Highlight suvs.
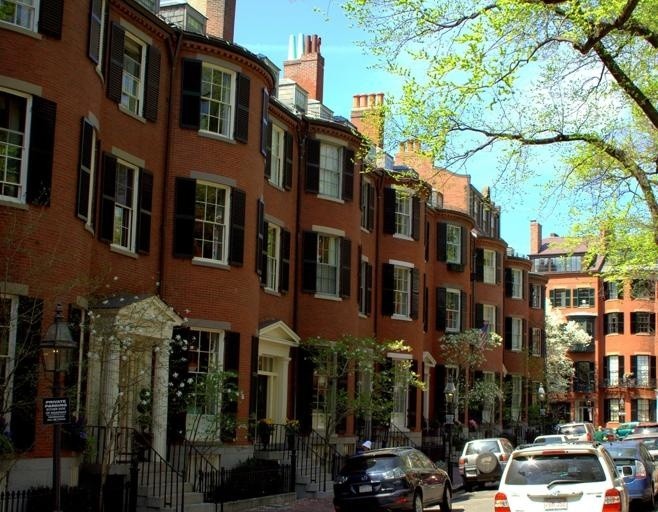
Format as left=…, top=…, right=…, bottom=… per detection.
left=492, top=440, right=633, bottom=512
left=456, top=436, right=518, bottom=494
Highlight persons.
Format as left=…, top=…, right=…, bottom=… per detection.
left=593, top=424, right=607, bottom=442
left=354, top=438, right=373, bottom=457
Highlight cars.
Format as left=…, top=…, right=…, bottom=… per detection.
left=528, top=417, right=658, bottom=508
left=330, top=443, right=456, bottom=512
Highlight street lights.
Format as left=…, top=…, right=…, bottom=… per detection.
left=584, top=383, right=595, bottom=422
left=443, top=367, right=457, bottom=485
left=537, top=381, right=547, bottom=434
left=33, top=301, right=82, bottom=512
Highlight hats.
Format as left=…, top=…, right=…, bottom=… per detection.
left=362, top=441, right=372, bottom=450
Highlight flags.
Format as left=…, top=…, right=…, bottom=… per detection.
left=476, top=322, right=489, bottom=352
